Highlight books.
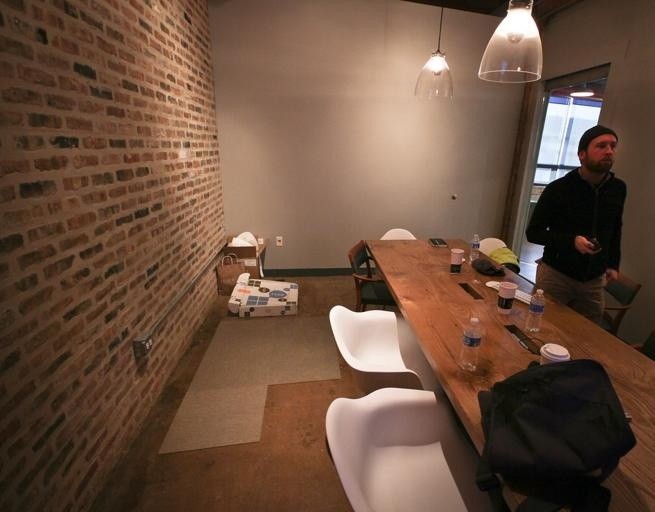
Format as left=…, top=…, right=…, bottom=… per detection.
left=429, top=238, right=448, bottom=247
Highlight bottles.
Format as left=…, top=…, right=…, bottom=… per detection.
left=525, top=289, right=545, bottom=332
left=470, top=234, right=480, bottom=261
left=459, top=317, right=481, bottom=371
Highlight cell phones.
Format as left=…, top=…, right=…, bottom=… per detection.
left=430, top=237, right=448, bottom=247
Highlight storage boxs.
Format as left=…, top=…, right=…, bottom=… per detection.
left=223, top=235, right=270, bottom=280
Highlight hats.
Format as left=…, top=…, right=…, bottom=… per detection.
left=578, top=125, right=618, bottom=156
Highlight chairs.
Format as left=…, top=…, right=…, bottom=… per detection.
left=379, top=227, right=417, bottom=240
left=479, top=237, right=507, bottom=256
left=630, top=329, right=654, bottom=360
left=329, top=304, right=442, bottom=393
left=603, top=271, right=642, bottom=335
left=348, top=239, right=397, bottom=312
left=325, top=388, right=508, bottom=511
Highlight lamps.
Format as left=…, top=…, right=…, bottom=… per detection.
left=478, top=0, right=543, bottom=84
left=414, top=0, right=454, bottom=100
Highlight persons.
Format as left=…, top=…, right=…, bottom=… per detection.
left=525, top=125, right=627, bottom=327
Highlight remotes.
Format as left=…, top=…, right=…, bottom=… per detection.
left=583, top=230, right=602, bottom=250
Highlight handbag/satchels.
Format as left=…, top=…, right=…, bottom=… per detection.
left=216, top=253, right=245, bottom=296
left=475, top=359, right=637, bottom=511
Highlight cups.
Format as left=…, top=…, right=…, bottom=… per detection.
left=497, top=282, right=518, bottom=315
left=449, top=248, right=464, bottom=273
left=539, top=343, right=570, bottom=366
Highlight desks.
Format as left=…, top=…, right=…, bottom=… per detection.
left=364, top=237, right=655, bottom=511
